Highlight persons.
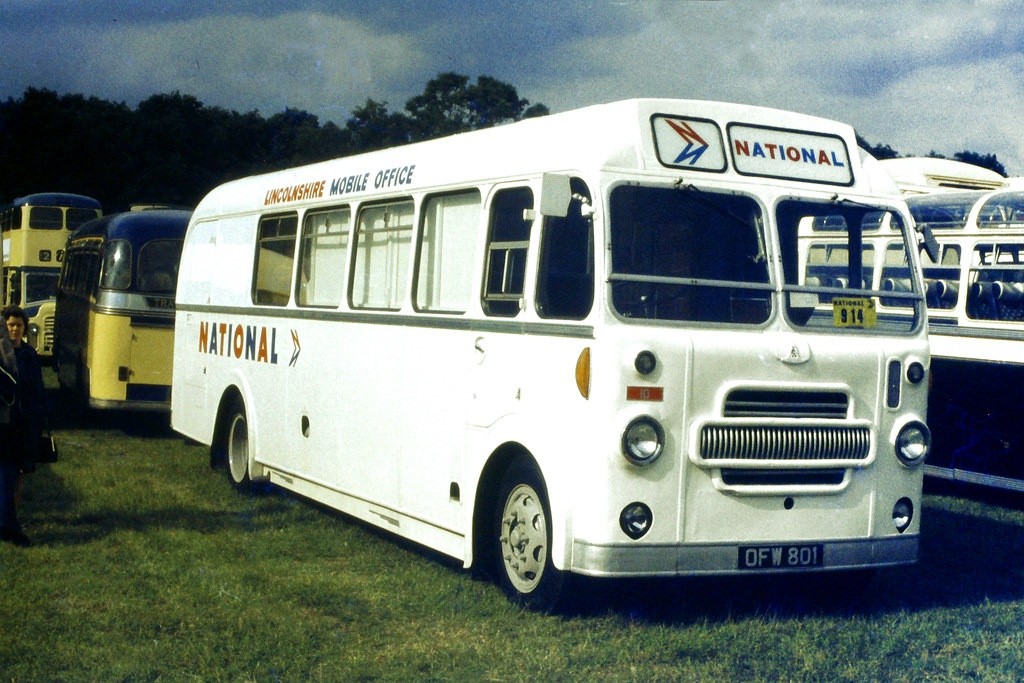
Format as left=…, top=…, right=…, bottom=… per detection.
left=0, top=306, right=58, bottom=548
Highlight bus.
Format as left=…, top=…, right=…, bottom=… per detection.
left=170, top=97, right=934, bottom=617
left=52, top=209, right=197, bottom=413
left=2, top=194, right=107, bottom=362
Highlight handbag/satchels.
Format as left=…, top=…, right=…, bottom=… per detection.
left=35, top=425, right=57, bottom=464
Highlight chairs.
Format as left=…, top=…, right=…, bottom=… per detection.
left=808, top=274, right=1024, bottom=324
left=142, top=270, right=174, bottom=291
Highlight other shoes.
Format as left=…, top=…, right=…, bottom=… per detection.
left=0, top=526, right=30, bottom=546
